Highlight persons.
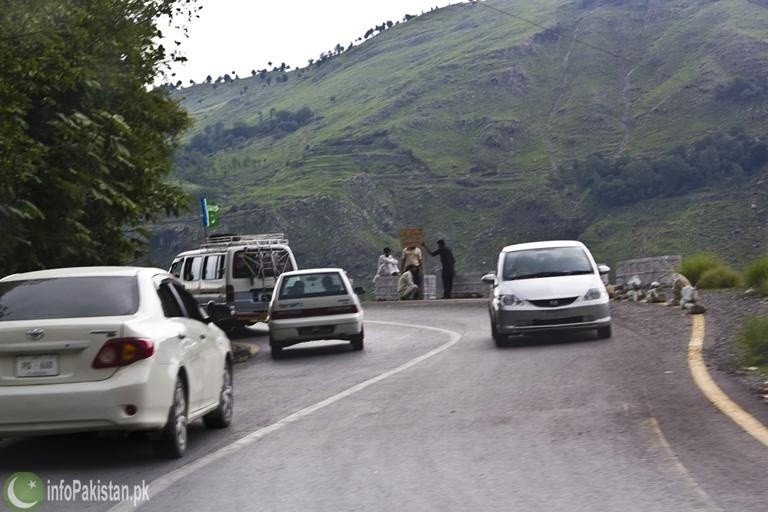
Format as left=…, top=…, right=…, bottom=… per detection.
left=421, top=240, right=456, bottom=299
left=378, top=247, right=401, bottom=275
left=397, top=247, right=424, bottom=300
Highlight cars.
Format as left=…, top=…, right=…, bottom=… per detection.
left=264, top=267, right=366, bottom=354
left=1, top=266, right=238, bottom=459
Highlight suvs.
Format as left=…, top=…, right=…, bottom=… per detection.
left=480, top=238, right=615, bottom=344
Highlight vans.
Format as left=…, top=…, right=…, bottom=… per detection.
left=168, top=233, right=299, bottom=335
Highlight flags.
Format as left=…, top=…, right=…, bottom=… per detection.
left=201, top=196, right=219, bottom=229
